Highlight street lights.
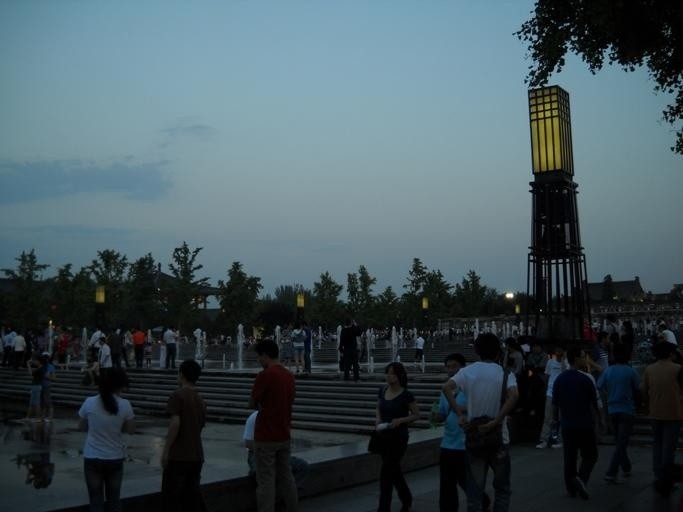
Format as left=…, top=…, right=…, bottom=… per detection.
left=297, top=290, right=305, bottom=326
left=420, top=297, right=431, bottom=334
left=93, top=283, right=106, bottom=330
left=515, top=302, right=521, bottom=328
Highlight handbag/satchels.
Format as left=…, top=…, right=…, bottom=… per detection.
left=464, top=416, right=502, bottom=447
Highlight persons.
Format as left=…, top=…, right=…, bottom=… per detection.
left=499, top=300, right=682, bottom=449
left=643, top=340, right=682, bottom=491
left=17, top=419, right=55, bottom=489
left=163, top=323, right=179, bottom=369
left=337, top=320, right=362, bottom=381
left=249, top=339, right=298, bottom=510
left=2, top=327, right=39, bottom=370
left=82, top=326, right=153, bottom=384
left=25, top=354, right=56, bottom=419
left=77, top=368, right=136, bottom=509
left=597, top=343, right=640, bottom=481
left=415, top=333, right=425, bottom=360
left=403, top=328, right=464, bottom=347
left=162, top=359, right=206, bottom=510
left=280, top=320, right=313, bottom=376
left=51, top=325, right=81, bottom=371
left=384, top=326, right=391, bottom=348
left=551, top=346, right=600, bottom=498
left=243, top=410, right=308, bottom=487
left=443, top=332, right=518, bottom=511
left=431, top=353, right=492, bottom=511
left=376, top=362, right=419, bottom=510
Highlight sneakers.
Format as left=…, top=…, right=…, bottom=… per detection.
left=605, top=469, right=631, bottom=480
left=567, top=475, right=589, bottom=498
left=536, top=435, right=561, bottom=448
